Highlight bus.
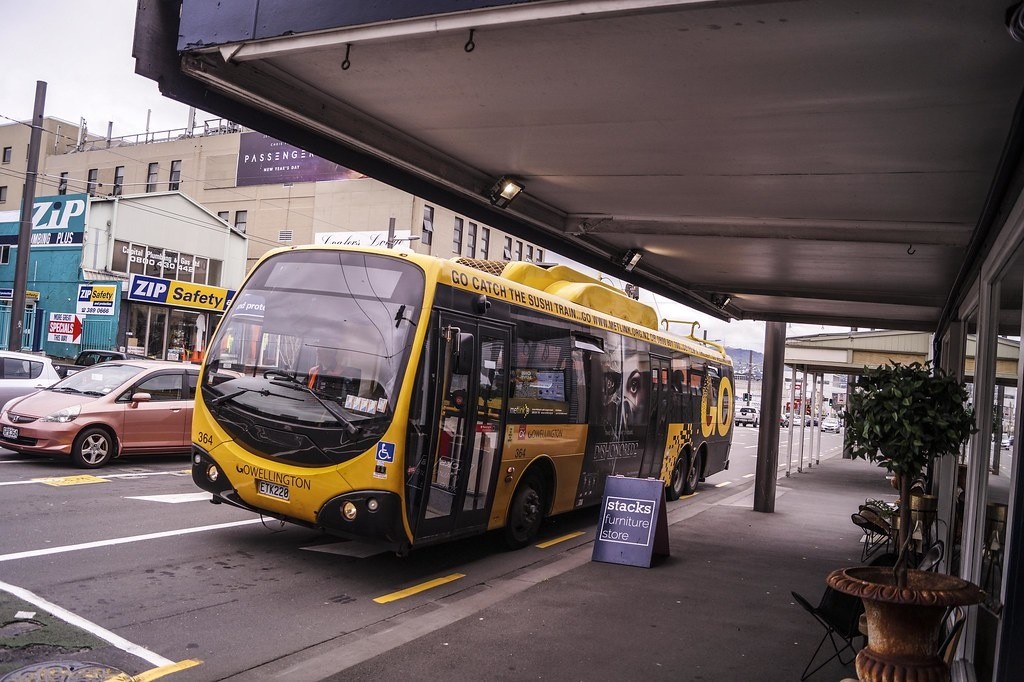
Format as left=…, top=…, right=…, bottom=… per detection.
left=190, top=243, right=738, bottom=560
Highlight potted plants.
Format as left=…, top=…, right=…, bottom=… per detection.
left=826, top=357, right=981, bottom=682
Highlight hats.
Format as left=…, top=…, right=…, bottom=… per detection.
left=314, top=337, right=339, bottom=347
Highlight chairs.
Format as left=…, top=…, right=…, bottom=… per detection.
left=340, top=364, right=360, bottom=399
left=156, top=377, right=177, bottom=400
left=792, top=540, right=966, bottom=682
left=851, top=498, right=892, bottom=563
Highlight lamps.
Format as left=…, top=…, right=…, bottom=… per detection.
left=712, top=292, right=731, bottom=309
left=476, top=176, right=526, bottom=210
left=611, top=249, right=643, bottom=272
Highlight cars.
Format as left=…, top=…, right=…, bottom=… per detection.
left=780, top=414, right=790, bottom=428
left=806, top=417, right=823, bottom=427
left=0, top=350, right=61, bottom=414
left=0, top=359, right=247, bottom=468
left=821, top=417, right=841, bottom=433
left=785, top=413, right=797, bottom=419
left=793, top=415, right=811, bottom=426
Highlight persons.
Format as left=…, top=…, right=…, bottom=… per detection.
left=602, top=332, right=650, bottom=426
left=301, top=334, right=356, bottom=399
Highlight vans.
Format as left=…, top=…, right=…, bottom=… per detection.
left=1001, top=433, right=1010, bottom=448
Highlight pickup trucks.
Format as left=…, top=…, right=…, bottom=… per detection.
left=52, top=349, right=157, bottom=380
left=734, top=407, right=760, bottom=427
